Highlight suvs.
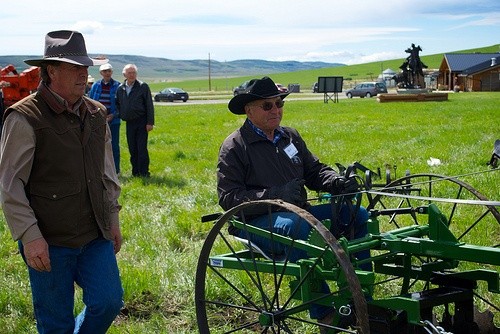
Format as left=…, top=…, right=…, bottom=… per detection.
left=346, top=82, right=388, bottom=99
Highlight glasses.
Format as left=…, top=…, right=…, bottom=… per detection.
left=250, top=99, right=286, bottom=111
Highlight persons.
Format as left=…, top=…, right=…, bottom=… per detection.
left=405, top=44, right=422, bottom=74
left=88, top=63, right=121, bottom=177
left=0, top=29, right=123, bottom=334
left=114, top=63, right=155, bottom=178
left=217, top=76, right=373, bottom=334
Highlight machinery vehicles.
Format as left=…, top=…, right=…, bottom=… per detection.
left=0, top=65, right=43, bottom=118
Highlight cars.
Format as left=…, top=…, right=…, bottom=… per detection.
left=311, top=82, right=319, bottom=93
left=154, top=88, right=189, bottom=102
left=275, top=83, right=289, bottom=92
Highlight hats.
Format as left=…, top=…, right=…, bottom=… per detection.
left=99, top=63, right=113, bottom=72
left=228, top=76, right=290, bottom=116
left=24, top=29, right=95, bottom=66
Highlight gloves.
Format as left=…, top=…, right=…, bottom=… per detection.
left=270, top=178, right=305, bottom=203
left=335, top=174, right=358, bottom=199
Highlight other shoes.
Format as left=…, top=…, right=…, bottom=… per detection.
left=319, top=309, right=352, bottom=334
